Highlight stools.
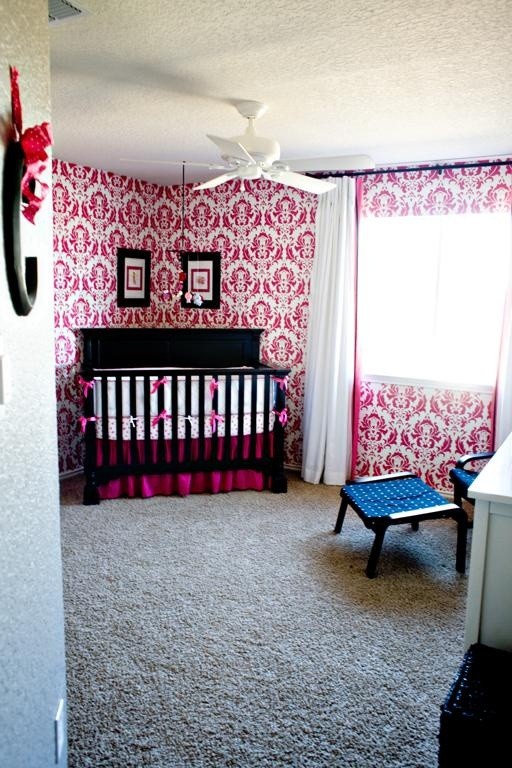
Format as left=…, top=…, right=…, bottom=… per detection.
left=334, top=471, right=468, bottom=578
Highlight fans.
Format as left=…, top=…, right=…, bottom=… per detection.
left=190, top=97, right=337, bottom=196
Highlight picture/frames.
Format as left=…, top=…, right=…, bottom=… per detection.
left=116, top=246, right=152, bottom=308
left=181, top=252, right=221, bottom=309
